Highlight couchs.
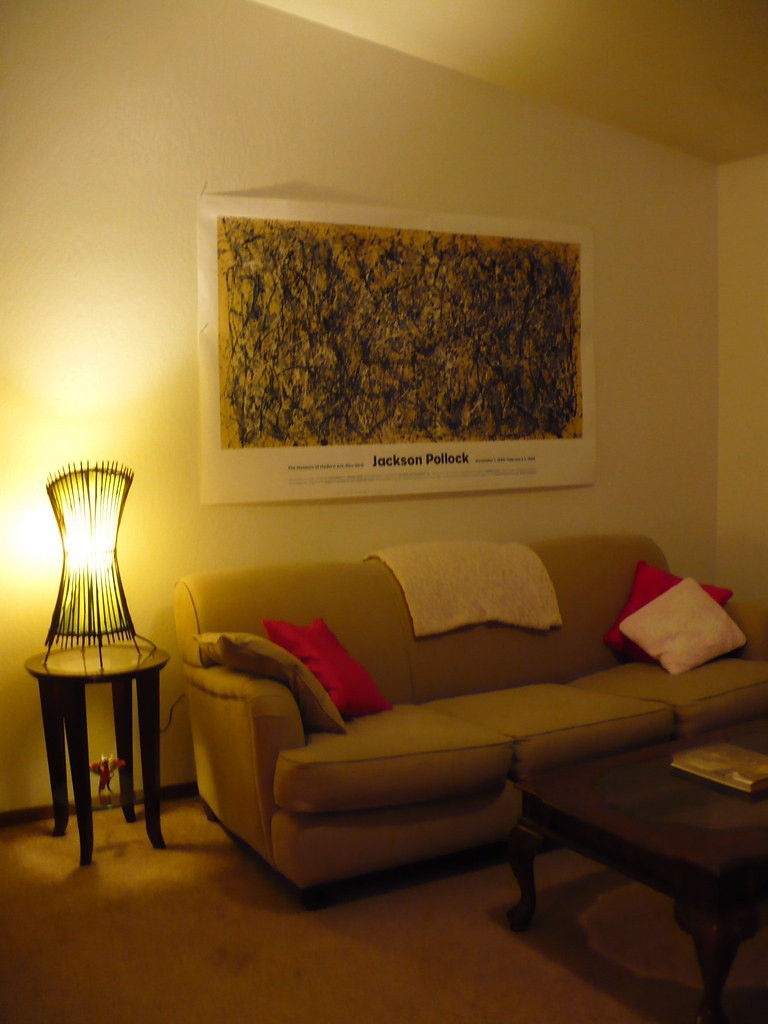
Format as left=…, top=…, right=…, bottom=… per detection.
left=167, top=532, right=768, bottom=909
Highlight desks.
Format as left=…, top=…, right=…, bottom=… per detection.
left=25, top=643, right=171, bottom=867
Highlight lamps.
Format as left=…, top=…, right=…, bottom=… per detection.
left=43, top=463, right=140, bottom=669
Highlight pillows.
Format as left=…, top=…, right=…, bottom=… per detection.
left=193, top=631, right=347, bottom=732
left=260, top=617, right=393, bottom=715
left=606, top=560, right=734, bottom=663
left=619, top=577, right=746, bottom=676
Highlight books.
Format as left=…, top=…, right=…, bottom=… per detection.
left=671, top=744, right=768, bottom=793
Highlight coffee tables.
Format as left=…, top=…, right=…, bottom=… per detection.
left=509, top=718, right=768, bottom=1023
